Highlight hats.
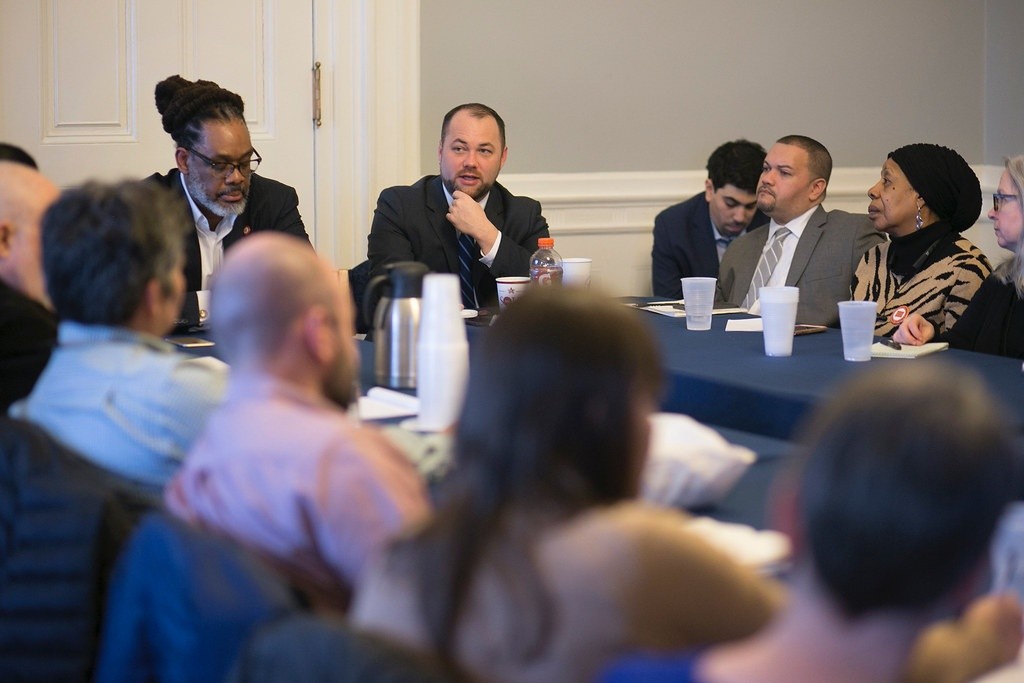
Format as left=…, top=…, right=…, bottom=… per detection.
left=887, top=143, right=982, bottom=233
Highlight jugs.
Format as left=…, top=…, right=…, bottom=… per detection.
left=361, top=259, right=430, bottom=389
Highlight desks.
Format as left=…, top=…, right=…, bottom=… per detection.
left=161, top=295, right=1024, bottom=536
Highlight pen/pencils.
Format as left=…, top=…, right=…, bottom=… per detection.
left=635, top=303, right=679, bottom=306
left=878, top=338, right=903, bottom=351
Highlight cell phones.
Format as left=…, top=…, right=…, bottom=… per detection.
left=464, top=317, right=490, bottom=326
left=165, top=337, right=215, bottom=347
left=793, top=324, right=827, bottom=335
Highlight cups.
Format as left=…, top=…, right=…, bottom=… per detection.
left=416, top=273, right=471, bottom=427
left=681, top=277, right=717, bottom=331
left=758, top=284, right=800, bottom=358
left=559, top=258, right=592, bottom=290
left=838, top=301, right=878, bottom=362
left=196, top=290, right=211, bottom=323
left=496, top=276, right=531, bottom=310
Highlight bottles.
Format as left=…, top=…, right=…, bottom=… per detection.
left=530, top=238, right=564, bottom=289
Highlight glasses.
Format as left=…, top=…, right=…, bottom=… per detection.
left=993, top=193, right=1024, bottom=211
left=182, top=143, right=263, bottom=176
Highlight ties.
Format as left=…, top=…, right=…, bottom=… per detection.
left=741, top=226, right=792, bottom=311
left=458, top=228, right=478, bottom=309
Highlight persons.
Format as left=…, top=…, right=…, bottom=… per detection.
left=850, top=143, right=993, bottom=338
left=7, top=179, right=231, bottom=487
left=164, top=231, right=436, bottom=632
left=651, top=139, right=771, bottom=301
left=342, top=279, right=1024, bottom=683
left=578, top=359, right=1024, bottom=683
left=713, top=135, right=888, bottom=328
left=142, top=75, right=317, bottom=334
left=367, top=103, right=550, bottom=310
left=0, top=143, right=64, bottom=413
left=892, top=155, right=1024, bottom=361
